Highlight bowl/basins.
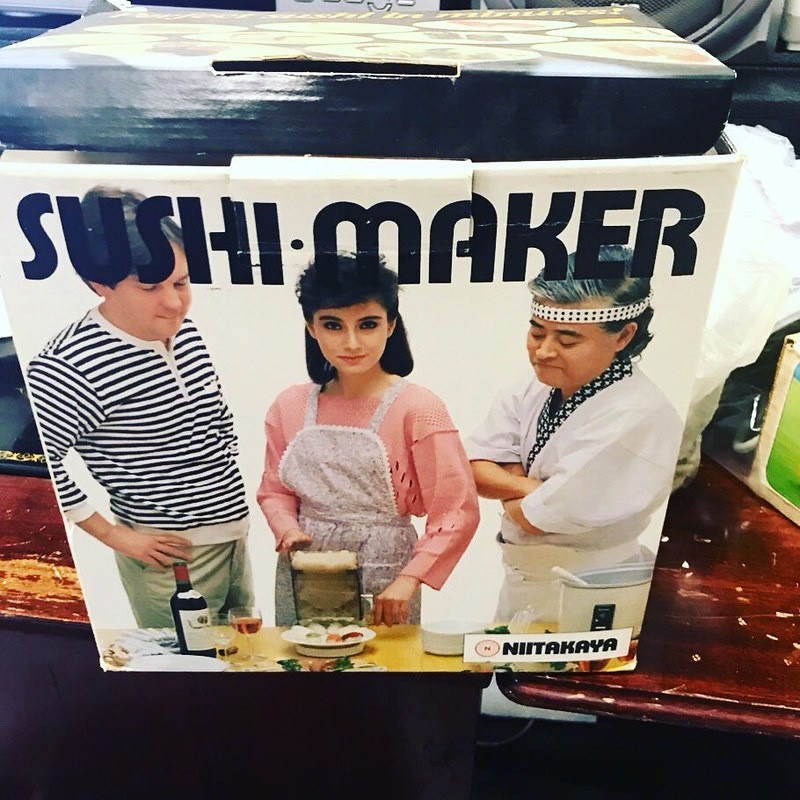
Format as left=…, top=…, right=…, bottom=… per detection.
left=420, top=620, right=488, bottom=656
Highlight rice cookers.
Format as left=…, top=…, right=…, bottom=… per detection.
left=558, top=561, right=656, bottom=631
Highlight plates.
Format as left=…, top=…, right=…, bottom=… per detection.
left=281, top=629, right=377, bottom=658
left=128, top=655, right=227, bottom=672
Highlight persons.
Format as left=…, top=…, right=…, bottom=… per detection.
left=463, top=247, right=679, bottom=625
left=25, top=184, right=257, bottom=629
left=257, top=254, right=481, bottom=627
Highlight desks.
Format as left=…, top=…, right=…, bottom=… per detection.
left=0, top=473, right=492, bottom=691
left=495, top=453, right=800, bottom=742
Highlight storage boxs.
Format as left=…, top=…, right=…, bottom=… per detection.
left=0, top=4, right=800, bottom=672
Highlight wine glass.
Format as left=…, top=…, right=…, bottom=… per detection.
left=203, top=613, right=244, bottom=667
left=228, top=606, right=268, bottom=662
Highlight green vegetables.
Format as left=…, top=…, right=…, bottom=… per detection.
left=484, top=626, right=569, bottom=671
left=277, top=656, right=354, bottom=672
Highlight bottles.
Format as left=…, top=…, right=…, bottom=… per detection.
left=169, top=561, right=217, bottom=659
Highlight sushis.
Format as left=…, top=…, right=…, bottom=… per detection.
left=306, top=631, right=363, bottom=644
